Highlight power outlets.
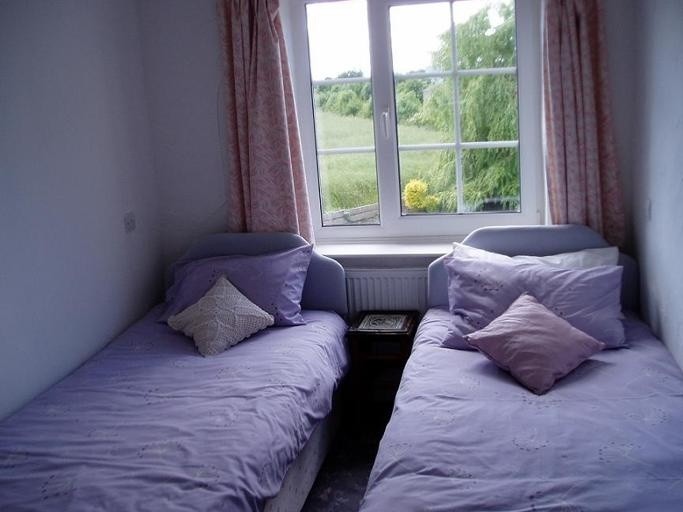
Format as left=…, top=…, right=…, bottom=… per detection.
left=124, top=212, right=136, bottom=234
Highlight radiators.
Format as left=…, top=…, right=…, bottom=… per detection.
left=346, top=266, right=428, bottom=313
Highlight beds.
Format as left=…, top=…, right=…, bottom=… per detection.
left=359, top=223, right=683, bottom=512
left=0, top=232, right=348, bottom=512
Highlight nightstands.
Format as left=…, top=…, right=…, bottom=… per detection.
left=347, top=309, right=420, bottom=408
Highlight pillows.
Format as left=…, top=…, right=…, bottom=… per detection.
left=157, top=243, right=313, bottom=327
left=441, top=256, right=628, bottom=348
left=462, top=290, right=605, bottom=395
left=167, top=276, right=275, bottom=359
left=452, top=241, right=619, bottom=267
